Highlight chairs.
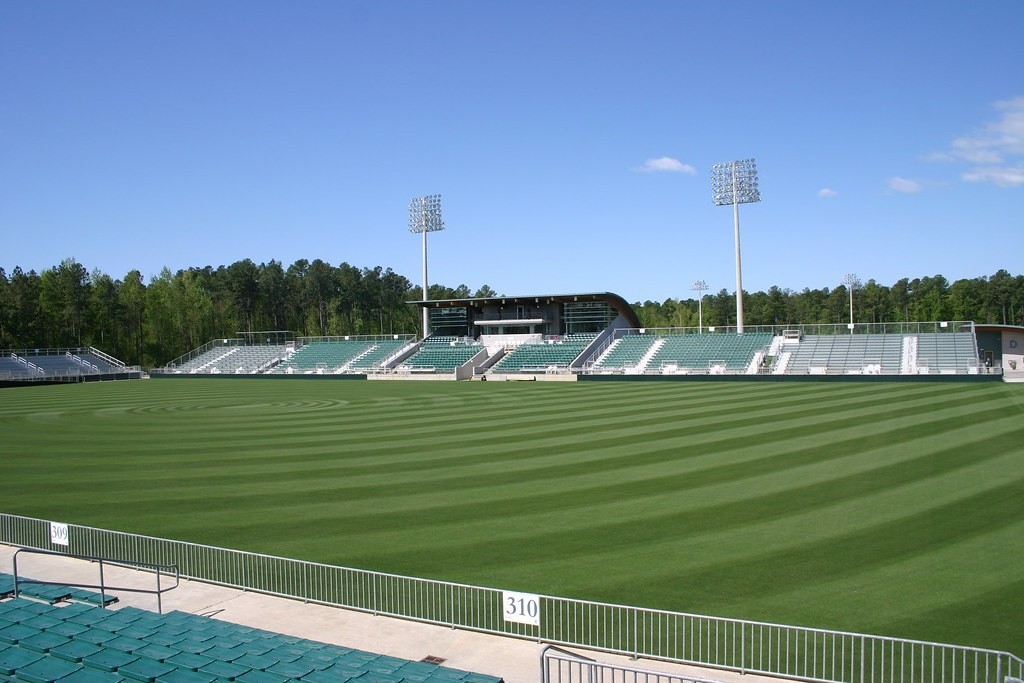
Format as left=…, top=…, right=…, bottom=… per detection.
left=165, top=330, right=775, bottom=379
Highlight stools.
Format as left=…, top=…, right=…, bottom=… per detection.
left=0, top=573, right=504, bottom=683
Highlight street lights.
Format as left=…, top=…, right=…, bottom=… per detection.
left=841, top=270, right=863, bottom=333
left=689, top=280, right=711, bottom=335
left=407, top=194, right=446, bottom=337
left=711, top=157, right=763, bottom=334
